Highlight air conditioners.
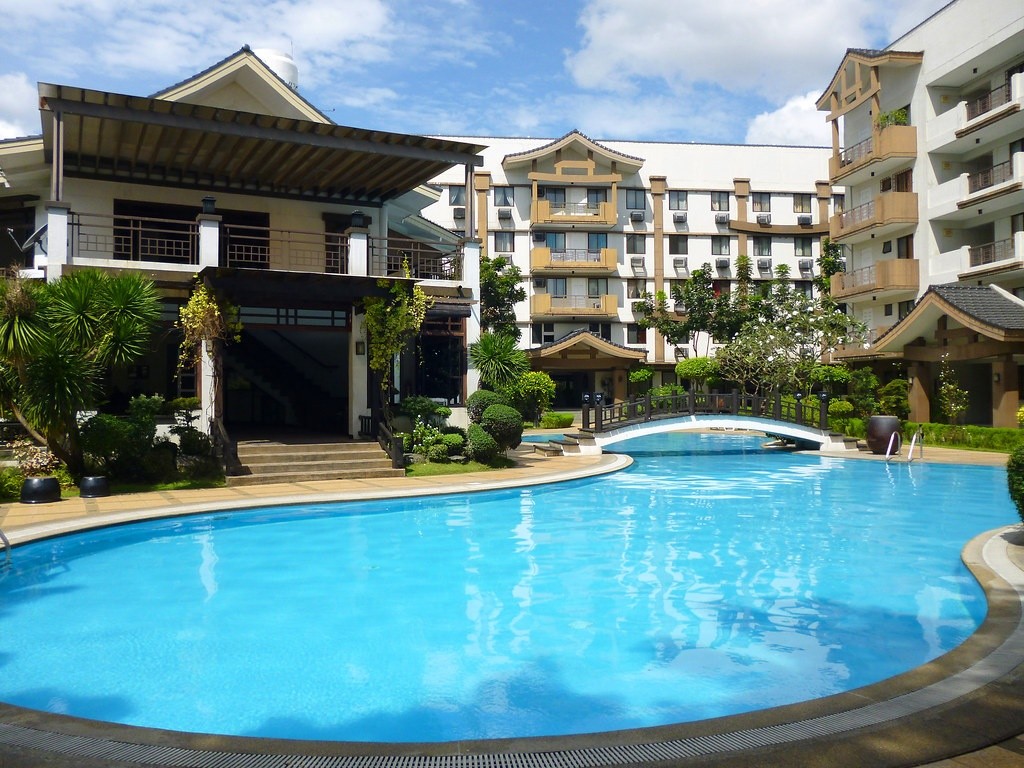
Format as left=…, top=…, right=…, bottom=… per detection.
left=673, top=257, right=687, bottom=268
left=799, top=258, right=813, bottom=269
left=630, top=210, right=644, bottom=222
left=533, top=278, right=545, bottom=287
left=715, top=212, right=730, bottom=224
left=757, top=214, right=771, bottom=223
left=454, top=207, right=465, bottom=219
left=499, top=254, right=512, bottom=265
left=673, top=212, right=687, bottom=223
left=498, top=208, right=511, bottom=220
left=631, top=256, right=645, bottom=267
left=532, top=232, right=545, bottom=242
left=757, top=258, right=772, bottom=269
left=715, top=257, right=730, bottom=268
left=797, top=215, right=812, bottom=225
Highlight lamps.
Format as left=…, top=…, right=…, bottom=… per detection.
left=355, top=341, right=366, bottom=356
